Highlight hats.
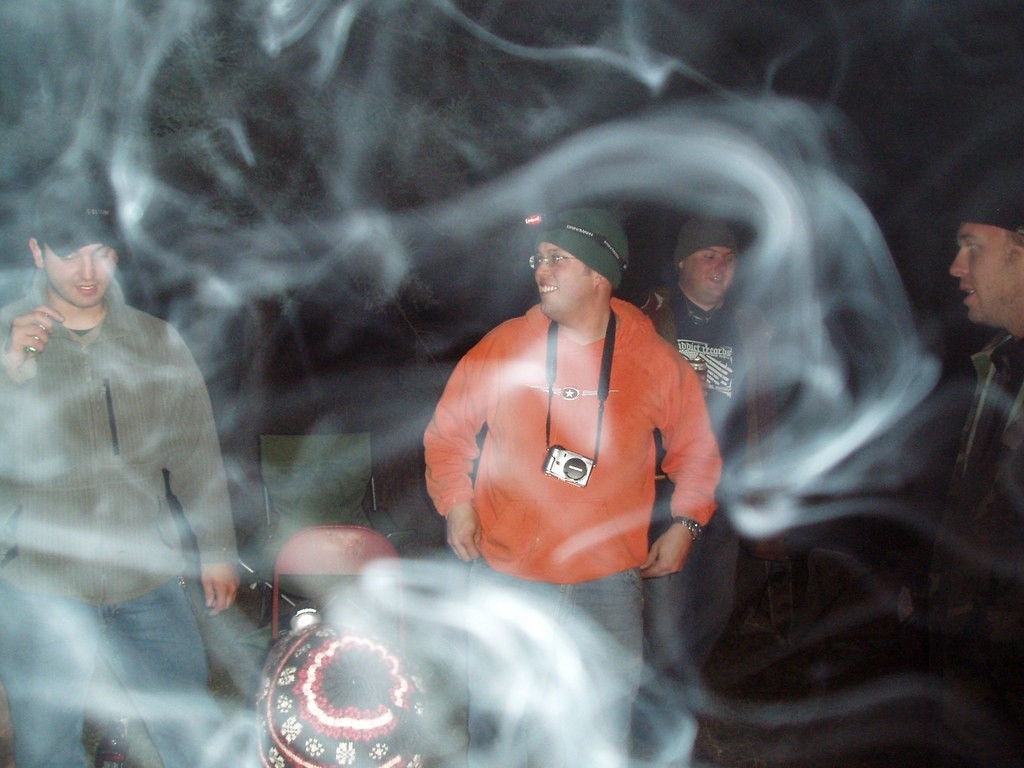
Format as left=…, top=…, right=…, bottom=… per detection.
left=671, top=215, right=738, bottom=266
left=31, top=178, right=126, bottom=257
left=951, top=174, right=1024, bottom=237
left=532, top=207, right=630, bottom=291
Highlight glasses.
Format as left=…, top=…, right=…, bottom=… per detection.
left=528, top=252, right=577, bottom=268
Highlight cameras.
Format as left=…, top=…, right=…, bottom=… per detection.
left=544, top=445, right=594, bottom=488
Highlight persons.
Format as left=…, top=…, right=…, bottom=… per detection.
left=251, top=158, right=1024, bottom=768
left=1, top=178, right=242, bottom=768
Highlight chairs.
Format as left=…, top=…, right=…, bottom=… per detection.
left=260, top=432, right=435, bottom=768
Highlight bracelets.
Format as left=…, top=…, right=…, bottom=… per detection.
left=671, top=516, right=704, bottom=542
